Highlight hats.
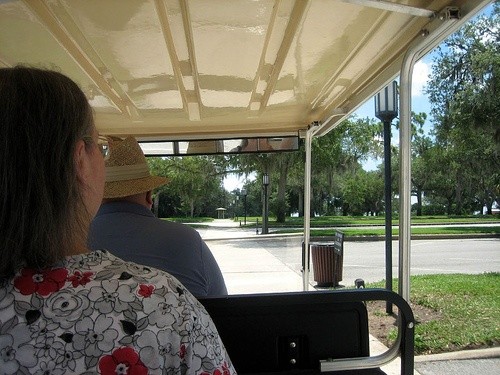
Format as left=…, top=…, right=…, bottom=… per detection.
left=101, top=136, right=171, bottom=200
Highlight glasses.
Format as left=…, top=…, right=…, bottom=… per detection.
left=80, top=136, right=114, bottom=160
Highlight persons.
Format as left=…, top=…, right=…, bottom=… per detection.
left=0, top=64, right=237, bottom=375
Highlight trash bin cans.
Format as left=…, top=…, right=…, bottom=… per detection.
left=311, top=242, right=343, bottom=288
